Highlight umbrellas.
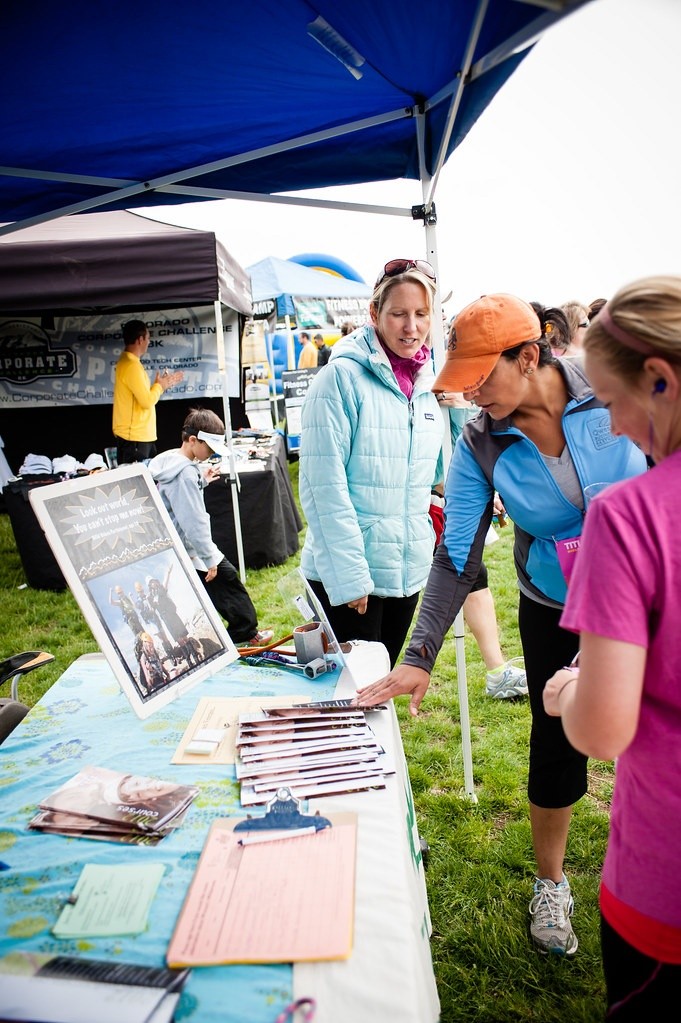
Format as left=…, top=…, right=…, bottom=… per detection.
left=244, top=252, right=369, bottom=370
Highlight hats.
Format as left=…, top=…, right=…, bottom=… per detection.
left=84, top=453, right=108, bottom=471
left=16, top=453, right=90, bottom=485
left=145, top=576, right=159, bottom=589
left=141, top=633, right=153, bottom=642
left=431, top=293, right=542, bottom=396
left=135, top=582, right=143, bottom=591
left=184, top=426, right=231, bottom=456
left=115, top=586, right=123, bottom=594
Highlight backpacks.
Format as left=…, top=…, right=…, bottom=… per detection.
left=140, top=649, right=164, bottom=687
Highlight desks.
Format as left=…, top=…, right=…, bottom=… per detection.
left=2, top=430, right=303, bottom=593
left=0, top=641, right=441, bottom=1023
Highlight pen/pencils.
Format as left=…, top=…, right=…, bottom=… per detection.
left=237, top=825, right=327, bottom=846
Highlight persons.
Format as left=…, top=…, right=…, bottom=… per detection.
left=545, top=276, right=681, bottom=1022
left=113, top=321, right=185, bottom=466
left=104, top=770, right=182, bottom=805
left=295, top=298, right=612, bottom=698
left=352, top=294, right=653, bottom=953
left=148, top=409, right=274, bottom=646
left=109, top=563, right=203, bottom=690
left=298, top=260, right=452, bottom=671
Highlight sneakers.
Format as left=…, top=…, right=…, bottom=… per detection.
left=234, top=628, right=274, bottom=646
left=532, top=871, right=579, bottom=954
left=483, top=664, right=530, bottom=699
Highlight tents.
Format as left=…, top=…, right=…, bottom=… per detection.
left=1, top=0, right=595, bottom=803
left=1, top=211, right=252, bottom=586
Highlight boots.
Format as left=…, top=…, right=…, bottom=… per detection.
left=180, top=641, right=198, bottom=667
left=161, top=641, right=176, bottom=667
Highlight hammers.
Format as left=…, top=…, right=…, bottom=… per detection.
left=261, top=656, right=326, bottom=679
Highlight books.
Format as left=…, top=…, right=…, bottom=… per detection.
left=25, top=766, right=202, bottom=846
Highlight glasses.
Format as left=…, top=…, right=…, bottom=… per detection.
left=577, top=319, right=590, bottom=327
left=373, top=259, right=437, bottom=289
